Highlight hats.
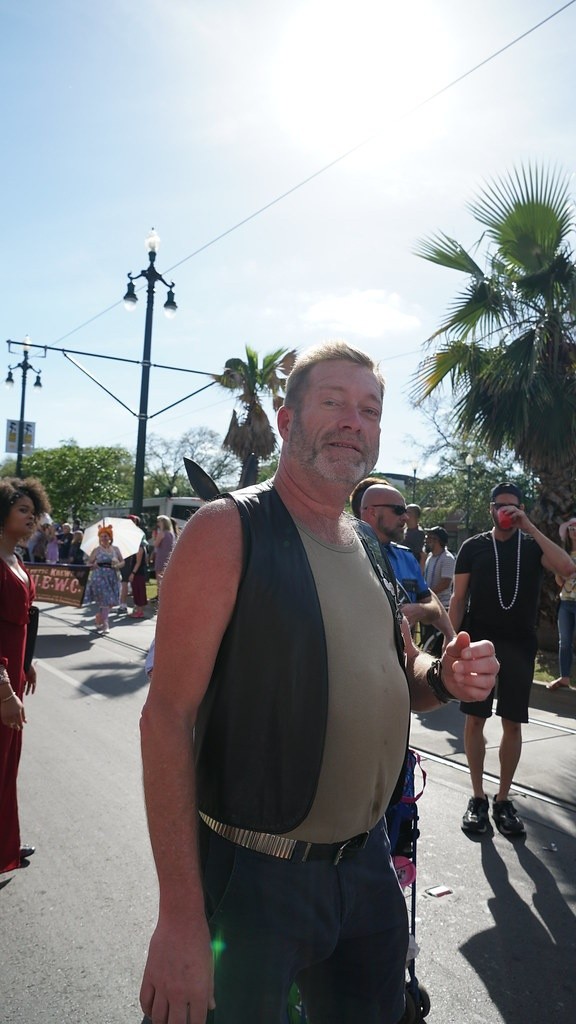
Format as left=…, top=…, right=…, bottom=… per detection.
left=97, top=524, right=114, bottom=546
left=559, top=517, right=576, bottom=541
left=424, top=525, right=447, bottom=543
left=493, top=482, right=521, bottom=502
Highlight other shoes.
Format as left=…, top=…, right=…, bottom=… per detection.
left=20, top=845, right=35, bottom=857
left=129, top=611, right=145, bottom=618
left=104, top=621, right=109, bottom=633
left=115, top=607, right=128, bottom=613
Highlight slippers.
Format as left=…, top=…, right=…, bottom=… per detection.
left=546, top=676, right=568, bottom=689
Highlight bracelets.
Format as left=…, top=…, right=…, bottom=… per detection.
left=2, top=692, right=16, bottom=702
left=426, top=659, right=457, bottom=705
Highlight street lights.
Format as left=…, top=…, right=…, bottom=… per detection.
left=121, top=224, right=179, bottom=517
left=411, top=456, right=420, bottom=505
left=3, top=335, right=43, bottom=480
left=464, top=451, right=475, bottom=544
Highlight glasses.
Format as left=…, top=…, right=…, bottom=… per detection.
left=364, top=505, right=407, bottom=515
left=567, top=525, right=576, bottom=531
left=494, top=503, right=520, bottom=510
left=424, top=534, right=440, bottom=541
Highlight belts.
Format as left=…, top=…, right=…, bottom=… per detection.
left=200, top=809, right=371, bottom=866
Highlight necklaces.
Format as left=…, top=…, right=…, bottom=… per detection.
left=492, top=527, right=521, bottom=609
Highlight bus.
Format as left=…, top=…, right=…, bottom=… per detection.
left=85, top=496, right=210, bottom=547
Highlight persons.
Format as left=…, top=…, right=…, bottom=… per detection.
left=135, top=345, right=503, bottom=1023
left=545, top=517, right=575, bottom=690
left=83, top=524, right=126, bottom=634
left=362, top=486, right=441, bottom=626
left=350, top=478, right=458, bottom=649
left=23, top=515, right=175, bottom=619
left=0, top=475, right=50, bottom=871
left=442, top=481, right=576, bottom=840
left=404, top=504, right=425, bottom=563
left=420, top=525, right=455, bottom=658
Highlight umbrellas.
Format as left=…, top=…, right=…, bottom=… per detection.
left=79, top=514, right=146, bottom=560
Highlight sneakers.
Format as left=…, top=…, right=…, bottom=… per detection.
left=461, top=795, right=488, bottom=833
left=492, top=794, right=524, bottom=835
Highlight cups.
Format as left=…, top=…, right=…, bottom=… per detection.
left=498, top=506, right=513, bottom=529
